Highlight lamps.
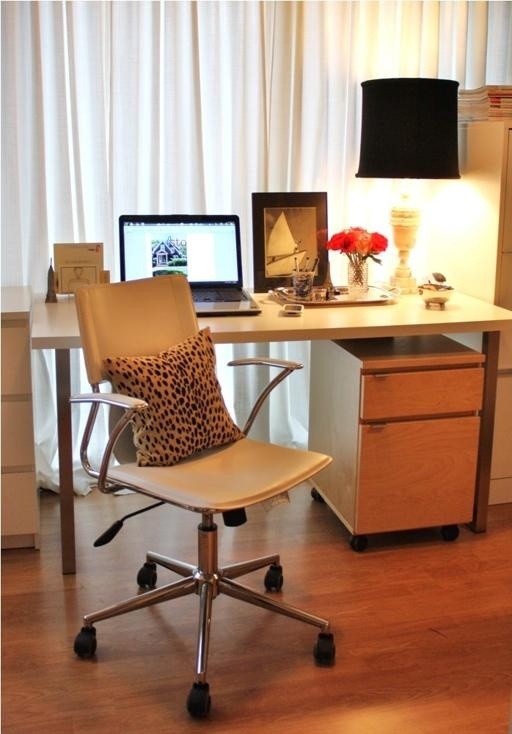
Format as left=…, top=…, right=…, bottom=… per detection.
left=350, top=73, right=463, bottom=300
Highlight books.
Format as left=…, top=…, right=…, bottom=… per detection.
left=458, top=85, right=512, bottom=127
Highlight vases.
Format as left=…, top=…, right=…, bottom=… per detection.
left=344, top=248, right=373, bottom=295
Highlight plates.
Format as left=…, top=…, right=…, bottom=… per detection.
left=274, top=287, right=397, bottom=304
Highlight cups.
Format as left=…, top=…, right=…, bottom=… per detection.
left=291, top=268, right=314, bottom=301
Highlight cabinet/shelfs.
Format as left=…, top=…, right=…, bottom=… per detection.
left=449, top=114, right=512, bottom=512
left=2, top=280, right=45, bottom=556
left=300, top=339, right=484, bottom=555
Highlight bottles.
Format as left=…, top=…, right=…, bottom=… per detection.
left=45, top=265, right=59, bottom=304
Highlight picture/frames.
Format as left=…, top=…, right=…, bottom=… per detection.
left=249, top=191, right=329, bottom=294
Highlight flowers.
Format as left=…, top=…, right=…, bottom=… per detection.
left=325, top=224, right=389, bottom=286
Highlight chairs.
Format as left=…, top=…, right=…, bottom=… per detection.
left=61, top=269, right=340, bottom=719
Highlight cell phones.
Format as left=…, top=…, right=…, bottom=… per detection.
left=282, top=304, right=304, bottom=317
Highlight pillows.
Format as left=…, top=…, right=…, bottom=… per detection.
left=102, top=321, right=246, bottom=469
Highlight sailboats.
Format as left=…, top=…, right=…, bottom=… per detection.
left=264, top=208, right=309, bottom=277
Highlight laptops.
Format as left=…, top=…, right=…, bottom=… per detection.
left=119, top=215, right=261, bottom=316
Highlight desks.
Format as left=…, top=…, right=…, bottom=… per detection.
left=27, top=283, right=512, bottom=581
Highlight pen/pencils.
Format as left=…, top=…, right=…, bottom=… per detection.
left=294, top=253, right=319, bottom=272
